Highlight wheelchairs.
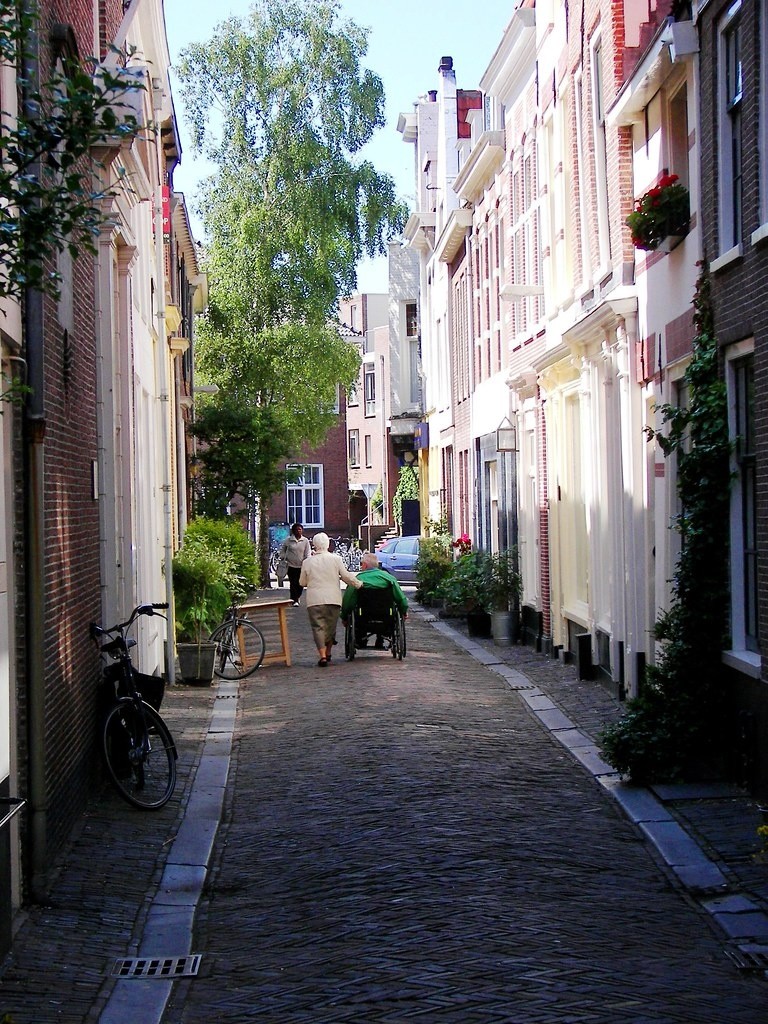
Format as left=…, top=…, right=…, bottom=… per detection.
left=345, top=586, right=406, bottom=661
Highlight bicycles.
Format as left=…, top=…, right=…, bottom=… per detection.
left=90, top=602, right=179, bottom=811
left=208, top=604, right=266, bottom=680
left=268, top=535, right=363, bottom=577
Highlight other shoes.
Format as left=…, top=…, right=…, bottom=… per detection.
left=293, top=602, right=299, bottom=607
left=375, top=642, right=383, bottom=647
left=354, top=638, right=367, bottom=648
left=298, top=597, right=301, bottom=604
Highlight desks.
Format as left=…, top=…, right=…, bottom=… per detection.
left=230, top=597, right=295, bottom=673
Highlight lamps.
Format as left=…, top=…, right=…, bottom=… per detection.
left=496, top=416, right=519, bottom=452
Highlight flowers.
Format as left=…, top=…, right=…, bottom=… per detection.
left=625, top=174, right=690, bottom=252
left=448, top=533, right=470, bottom=556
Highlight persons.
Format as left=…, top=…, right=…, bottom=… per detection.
left=280, top=523, right=312, bottom=607
left=299, top=533, right=363, bottom=667
left=340, top=554, right=410, bottom=647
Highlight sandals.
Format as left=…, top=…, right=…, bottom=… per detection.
left=327, top=654, right=332, bottom=661
left=318, top=657, right=327, bottom=667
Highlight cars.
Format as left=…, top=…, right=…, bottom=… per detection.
left=374, top=534, right=420, bottom=588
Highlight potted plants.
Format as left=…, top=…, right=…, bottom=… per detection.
left=425, top=546, right=524, bottom=648
left=161, top=530, right=248, bottom=685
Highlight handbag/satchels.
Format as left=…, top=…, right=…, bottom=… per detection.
left=277, top=559, right=287, bottom=578
left=133, top=666, right=165, bottom=728
